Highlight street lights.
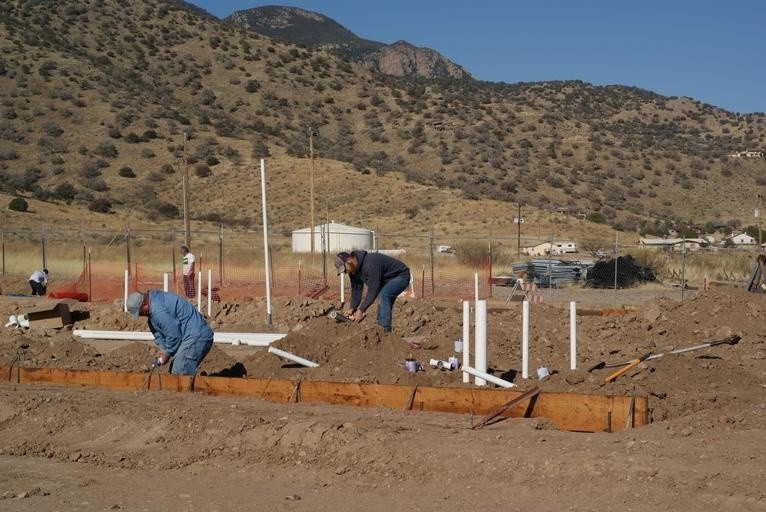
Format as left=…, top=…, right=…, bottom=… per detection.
left=755, top=206, right=762, bottom=252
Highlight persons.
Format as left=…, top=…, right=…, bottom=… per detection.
left=331, top=249, right=411, bottom=332
left=179, top=245, right=197, bottom=298
left=122, top=288, right=214, bottom=376
left=28, top=267, right=49, bottom=297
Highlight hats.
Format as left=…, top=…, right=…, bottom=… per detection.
left=334, top=252, right=347, bottom=276
left=126, top=292, right=144, bottom=322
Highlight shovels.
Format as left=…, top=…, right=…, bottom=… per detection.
left=588, top=335, right=742, bottom=373
